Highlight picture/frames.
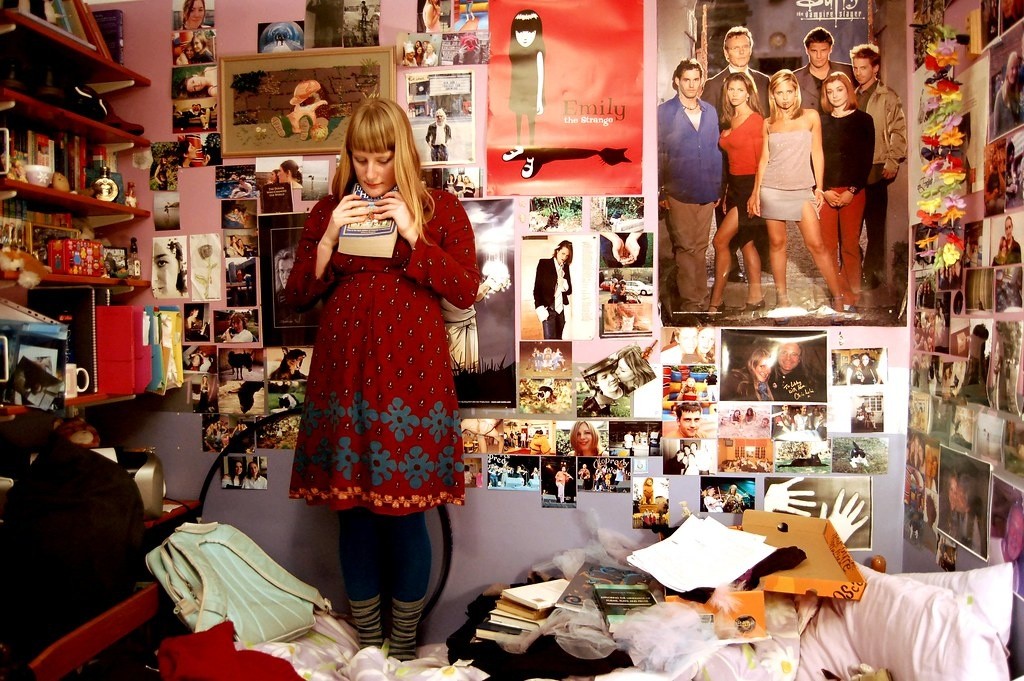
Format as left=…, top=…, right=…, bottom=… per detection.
left=212, top=43, right=402, bottom=162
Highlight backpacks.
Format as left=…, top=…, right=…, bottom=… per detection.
left=146, top=520, right=332, bottom=646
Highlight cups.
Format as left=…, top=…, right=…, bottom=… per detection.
left=64, top=363, right=89, bottom=399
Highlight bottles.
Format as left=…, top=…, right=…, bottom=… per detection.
left=91, top=165, right=120, bottom=204
left=126, top=237, right=142, bottom=280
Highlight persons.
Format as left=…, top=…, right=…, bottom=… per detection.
left=286, top=95, right=479, bottom=659
left=152, top=0, right=1024, bottom=601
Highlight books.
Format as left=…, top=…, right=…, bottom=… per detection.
left=0, top=0, right=148, bottom=397
left=337, top=183, right=399, bottom=258
left=475, top=560, right=660, bottom=646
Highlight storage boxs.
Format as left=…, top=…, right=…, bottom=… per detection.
left=660, top=506, right=866, bottom=640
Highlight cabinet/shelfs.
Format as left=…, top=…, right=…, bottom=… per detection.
left=0, top=9, right=159, bottom=424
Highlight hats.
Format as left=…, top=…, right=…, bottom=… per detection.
left=583, top=358, right=617, bottom=378
left=706, top=486, right=712, bottom=490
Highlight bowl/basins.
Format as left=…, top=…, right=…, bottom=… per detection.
left=24, top=164, right=53, bottom=188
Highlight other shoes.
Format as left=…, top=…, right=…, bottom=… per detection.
left=831, top=296, right=845, bottom=325
left=702, top=303, right=725, bottom=321
left=727, top=268, right=745, bottom=282
left=746, top=299, right=765, bottom=311
left=773, top=306, right=789, bottom=325
left=74, top=83, right=144, bottom=136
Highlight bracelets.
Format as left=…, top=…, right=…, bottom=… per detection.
left=815, top=189, right=825, bottom=195
left=848, top=186, right=859, bottom=194
